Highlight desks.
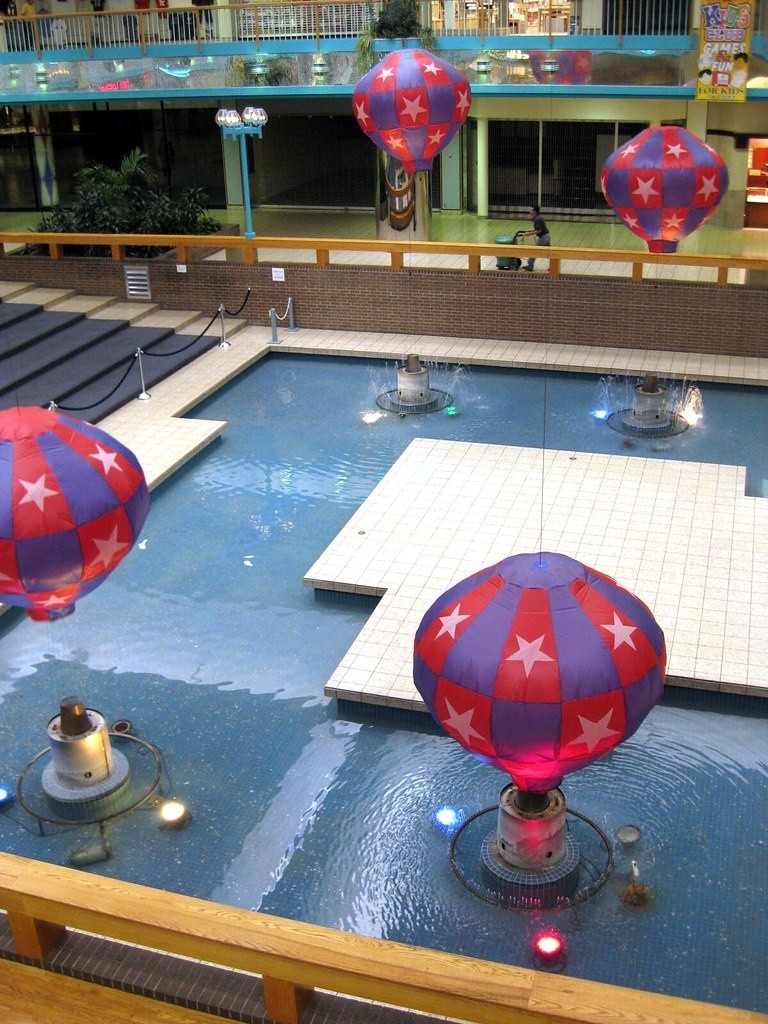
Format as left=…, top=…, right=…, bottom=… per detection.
left=460, top=5, right=569, bottom=35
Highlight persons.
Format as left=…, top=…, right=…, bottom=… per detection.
left=21, top=0, right=36, bottom=52
left=521, top=205, right=552, bottom=272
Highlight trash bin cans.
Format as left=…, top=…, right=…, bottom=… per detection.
left=495, top=234, right=517, bottom=268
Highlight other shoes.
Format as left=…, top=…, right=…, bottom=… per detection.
left=523, top=266, right=534, bottom=272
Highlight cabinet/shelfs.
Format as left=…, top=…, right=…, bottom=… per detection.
left=743, top=187, right=768, bottom=228
